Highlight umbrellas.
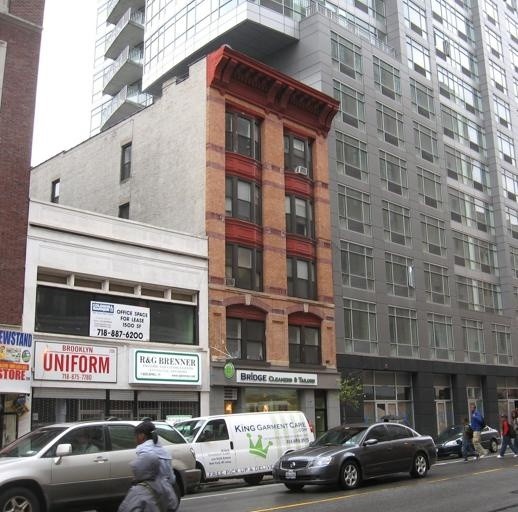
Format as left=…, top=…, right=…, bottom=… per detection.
left=380, top=414, right=402, bottom=421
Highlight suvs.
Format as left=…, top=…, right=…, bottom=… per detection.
left=0, top=421, right=202, bottom=511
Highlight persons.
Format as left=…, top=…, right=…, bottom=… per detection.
left=468, top=402, right=489, bottom=460
left=497, top=407, right=518, bottom=460
left=461, top=417, right=480, bottom=465
left=117, top=453, right=179, bottom=512
left=383, top=418, right=388, bottom=422
left=117, top=421, right=181, bottom=512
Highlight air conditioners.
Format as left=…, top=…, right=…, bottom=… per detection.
left=224, top=277, right=234, bottom=287
left=294, top=165, right=307, bottom=176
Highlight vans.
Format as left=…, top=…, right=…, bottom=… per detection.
left=171, top=409, right=314, bottom=489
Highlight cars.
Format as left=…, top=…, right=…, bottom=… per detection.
left=432, top=421, right=500, bottom=457
left=274, top=423, right=438, bottom=488
